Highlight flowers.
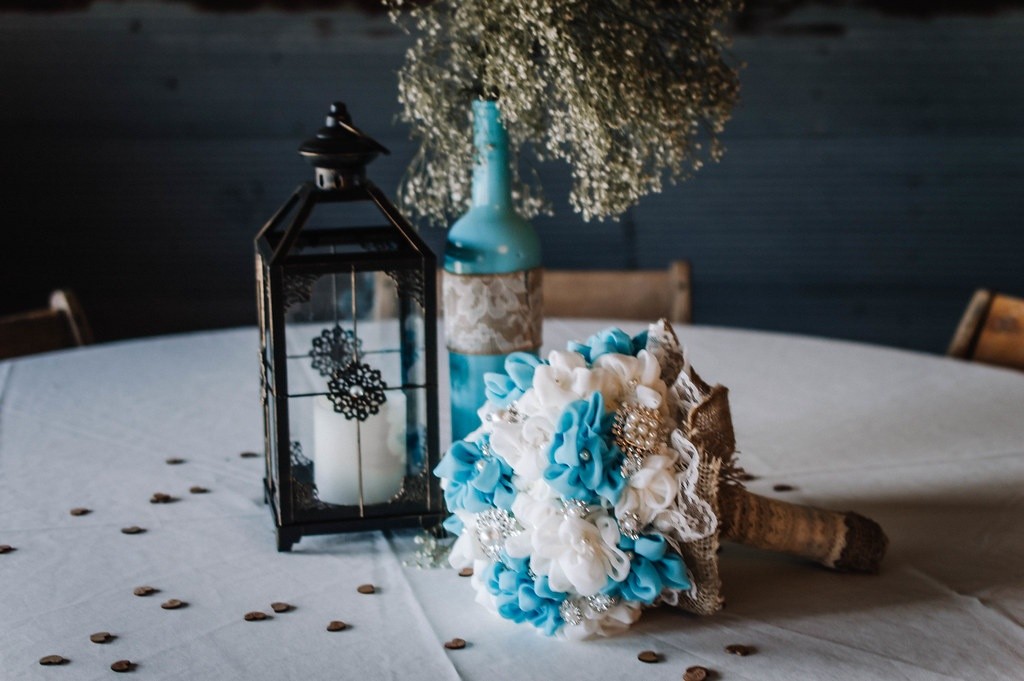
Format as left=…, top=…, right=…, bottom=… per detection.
left=400, top=321, right=890, bottom=642
left=381, top=0, right=747, bottom=230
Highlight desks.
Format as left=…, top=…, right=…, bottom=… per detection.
left=0, top=322, right=1024, bottom=681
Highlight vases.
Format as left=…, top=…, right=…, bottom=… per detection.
left=440, top=98, right=544, bottom=448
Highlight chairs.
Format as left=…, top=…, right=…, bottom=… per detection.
left=374, top=262, right=690, bottom=326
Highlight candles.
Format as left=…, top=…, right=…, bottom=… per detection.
left=314, top=390, right=408, bottom=506
